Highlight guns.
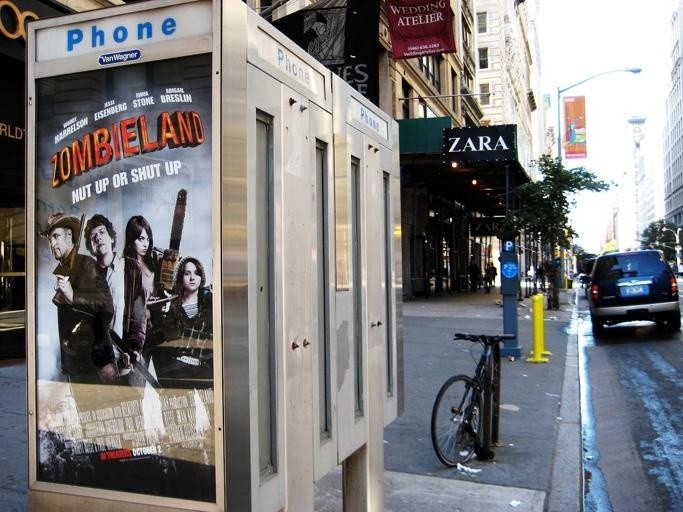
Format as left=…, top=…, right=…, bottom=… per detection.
left=53, top=212, right=85, bottom=307
left=147, top=284, right=213, bottom=308
left=109, top=329, right=162, bottom=394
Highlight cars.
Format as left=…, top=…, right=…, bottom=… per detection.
left=587, top=248, right=680, bottom=342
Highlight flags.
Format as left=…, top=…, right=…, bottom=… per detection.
left=385, top=1, right=456, bottom=61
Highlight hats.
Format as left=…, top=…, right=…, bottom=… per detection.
left=39, top=213, right=80, bottom=243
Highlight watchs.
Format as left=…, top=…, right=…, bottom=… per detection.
left=82, top=213, right=148, bottom=389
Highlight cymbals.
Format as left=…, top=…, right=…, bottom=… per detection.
left=156, top=337, right=213, bottom=349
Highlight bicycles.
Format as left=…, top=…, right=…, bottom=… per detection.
left=430, top=334, right=517, bottom=466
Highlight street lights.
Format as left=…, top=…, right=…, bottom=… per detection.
left=557, top=65, right=643, bottom=291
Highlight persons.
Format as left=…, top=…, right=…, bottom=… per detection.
left=484, top=264, right=492, bottom=293
left=121, top=214, right=182, bottom=430
left=489, top=263, right=496, bottom=287
left=162, top=255, right=213, bottom=469
left=304, top=22, right=326, bottom=53
left=468, top=259, right=480, bottom=293
left=37, top=211, right=125, bottom=439
